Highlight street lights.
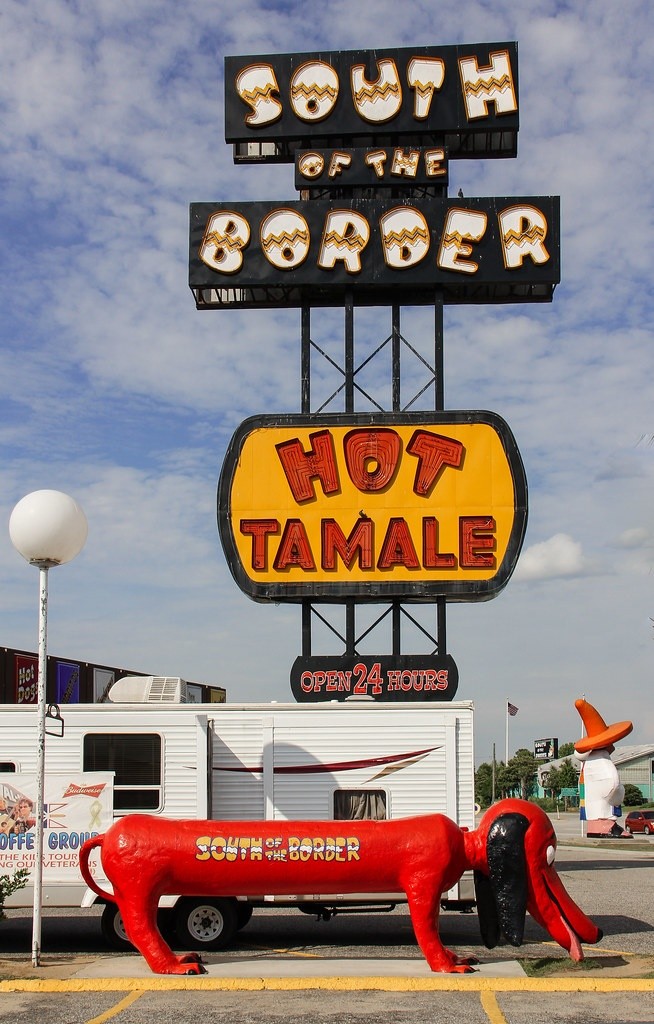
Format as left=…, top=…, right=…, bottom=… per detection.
left=7, top=488, right=89, bottom=968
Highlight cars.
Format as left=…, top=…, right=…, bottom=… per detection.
left=624, top=810, right=654, bottom=835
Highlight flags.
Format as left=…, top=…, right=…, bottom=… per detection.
left=508, top=702, right=519, bottom=716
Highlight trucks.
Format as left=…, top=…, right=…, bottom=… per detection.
left=0, top=676, right=477, bottom=953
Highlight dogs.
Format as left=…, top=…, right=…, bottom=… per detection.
left=78, top=797, right=604, bottom=977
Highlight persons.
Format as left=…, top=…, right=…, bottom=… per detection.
left=574, top=699, right=633, bottom=838
left=0, top=796, right=37, bottom=834
